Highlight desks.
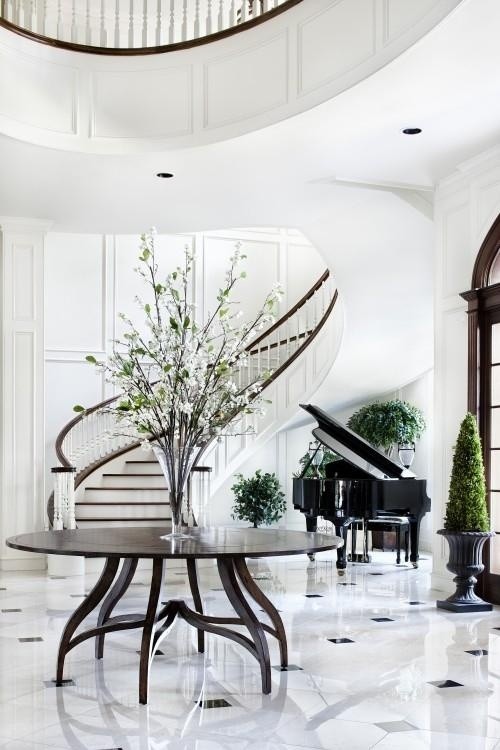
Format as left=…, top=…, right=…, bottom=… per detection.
left=4, top=524, right=346, bottom=705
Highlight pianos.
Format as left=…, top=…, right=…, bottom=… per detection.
left=293, top=404, right=431, bottom=576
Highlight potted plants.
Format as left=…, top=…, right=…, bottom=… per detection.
left=346, top=398, right=498, bottom=614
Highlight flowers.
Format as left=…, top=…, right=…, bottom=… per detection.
left=84, top=223, right=274, bottom=442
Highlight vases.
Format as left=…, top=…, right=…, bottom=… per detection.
left=145, top=442, right=203, bottom=540
